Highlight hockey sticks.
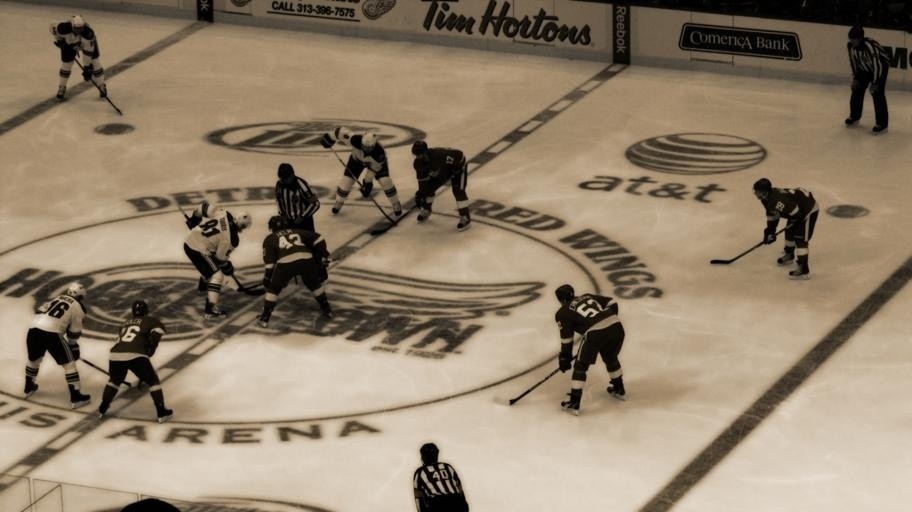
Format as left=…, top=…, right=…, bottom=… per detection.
left=369, top=185, right=450, bottom=235
left=710, top=224, right=794, bottom=266
left=71, top=54, right=123, bottom=116
left=493, top=356, right=576, bottom=406
left=175, top=204, right=256, bottom=291
left=77, top=357, right=144, bottom=389
left=330, top=147, right=401, bottom=227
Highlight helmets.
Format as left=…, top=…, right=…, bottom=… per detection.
left=70, top=14, right=86, bottom=29
left=67, top=282, right=87, bottom=300
left=277, top=162, right=294, bottom=181
left=753, top=178, right=772, bottom=193
left=132, top=299, right=151, bottom=318
left=268, top=214, right=283, bottom=231
left=555, top=284, right=574, bottom=304
left=360, top=130, right=377, bottom=149
left=232, top=210, right=253, bottom=231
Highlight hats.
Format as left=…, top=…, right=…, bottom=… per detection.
left=848, top=23, right=865, bottom=40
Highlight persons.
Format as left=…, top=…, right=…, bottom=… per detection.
left=845, top=25, right=889, bottom=132
left=554, top=284, right=625, bottom=408
left=411, top=139, right=470, bottom=229
left=22, top=282, right=91, bottom=403
left=318, top=126, right=402, bottom=216
left=99, top=299, right=172, bottom=417
left=412, top=442, right=472, bottom=510
left=752, top=179, right=819, bottom=278
left=183, top=202, right=251, bottom=317
left=49, top=15, right=106, bottom=97
left=274, top=162, right=321, bottom=231
left=255, top=215, right=332, bottom=323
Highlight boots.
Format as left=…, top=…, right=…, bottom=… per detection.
left=98, top=83, right=107, bottom=98
left=789, top=264, right=810, bottom=277
left=254, top=299, right=277, bottom=323
left=560, top=393, right=580, bottom=411
left=315, top=292, right=332, bottom=315
left=205, top=302, right=227, bottom=317
left=56, top=84, right=67, bottom=98
left=198, top=281, right=208, bottom=292
left=97, top=383, right=119, bottom=414
left=23, top=381, right=39, bottom=393
left=149, top=388, right=174, bottom=418
left=606, top=381, right=625, bottom=396
left=69, top=389, right=91, bottom=403
left=777, top=248, right=795, bottom=264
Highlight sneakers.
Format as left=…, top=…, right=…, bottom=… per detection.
left=872, top=122, right=888, bottom=131
left=456, top=215, right=470, bottom=228
left=416, top=206, right=432, bottom=221
left=845, top=116, right=861, bottom=124
left=392, top=200, right=402, bottom=215
left=331, top=201, right=344, bottom=214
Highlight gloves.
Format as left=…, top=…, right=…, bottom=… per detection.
left=557, top=352, right=571, bottom=374
left=320, top=132, right=336, bottom=148
left=359, top=178, right=373, bottom=198
left=68, top=342, right=81, bottom=361
left=186, top=210, right=203, bottom=230
left=81, top=63, right=94, bottom=81
left=764, top=228, right=777, bottom=245
left=869, top=81, right=879, bottom=95
left=850, top=79, right=860, bottom=92
